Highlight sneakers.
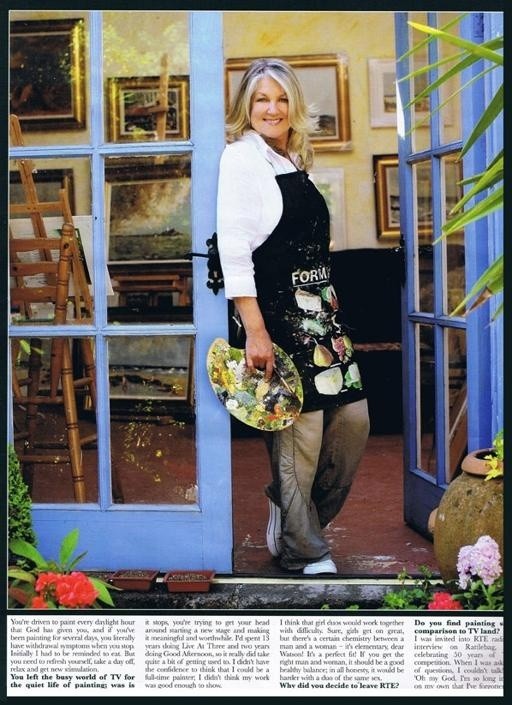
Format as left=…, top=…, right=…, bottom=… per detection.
left=267, top=499, right=284, bottom=557
left=303, top=560, right=336, bottom=575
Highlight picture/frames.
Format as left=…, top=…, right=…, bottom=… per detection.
left=224, top=54, right=467, bottom=251
left=6, top=18, right=196, bottom=407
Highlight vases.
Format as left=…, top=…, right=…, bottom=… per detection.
left=428, top=447, right=504, bottom=586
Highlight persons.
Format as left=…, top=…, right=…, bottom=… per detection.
left=216, top=56, right=375, bottom=583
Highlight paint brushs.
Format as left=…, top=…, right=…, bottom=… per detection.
left=230, top=315, right=301, bottom=401
